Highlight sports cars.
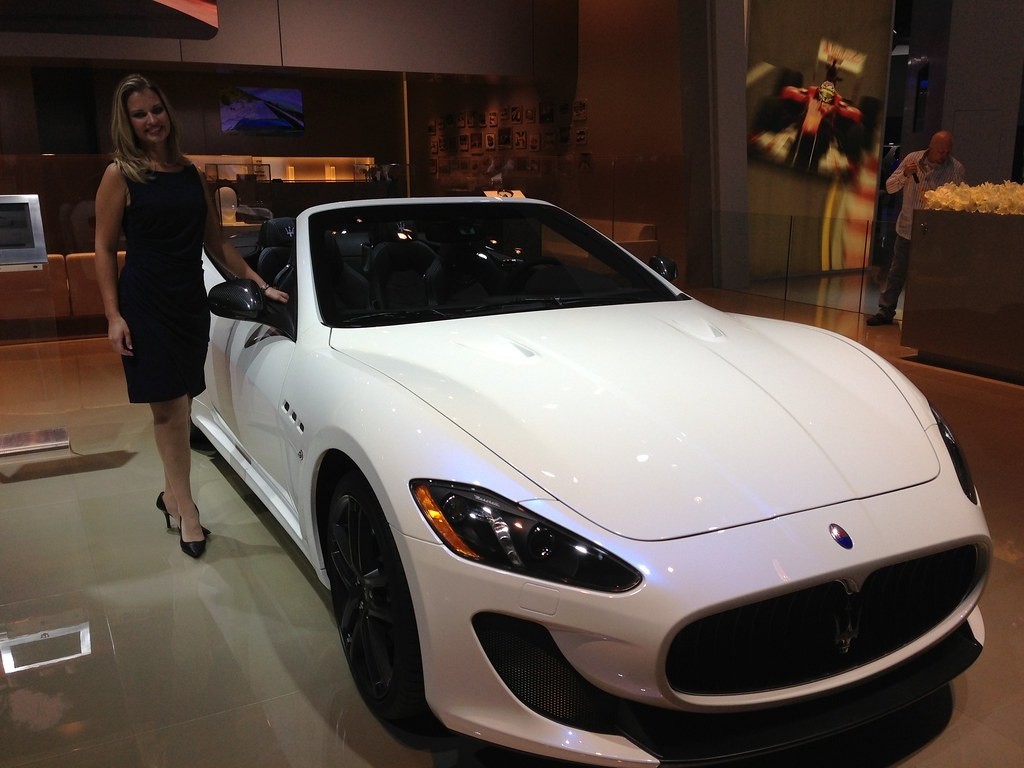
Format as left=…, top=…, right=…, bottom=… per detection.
left=190, top=195, right=990, bottom=768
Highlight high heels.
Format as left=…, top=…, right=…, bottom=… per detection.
left=179, top=503, right=206, bottom=558
left=156, top=491, right=211, bottom=536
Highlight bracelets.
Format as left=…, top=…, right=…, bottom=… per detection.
left=261, top=284, right=269, bottom=290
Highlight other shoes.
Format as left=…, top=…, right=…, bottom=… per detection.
left=867, top=313, right=893, bottom=326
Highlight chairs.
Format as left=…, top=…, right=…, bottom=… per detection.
left=256, top=217, right=446, bottom=320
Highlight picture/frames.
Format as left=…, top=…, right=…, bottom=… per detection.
left=573, top=127, right=587, bottom=145
left=529, top=134, right=541, bottom=152
left=529, top=159, right=543, bottom=177
left=512, top=128, right=528, bottom=151
left=525, top=109, right=535, bottom=124
left=510, top=105, right=523, bottom=125
left=425, top=110, right=498, bottom=174
left=573, top=100, right=587, bottom=121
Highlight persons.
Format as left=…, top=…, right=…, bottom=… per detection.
left=95, top=74, right=289, bottom=559
left=868, top=131, right=966, bottom=326
